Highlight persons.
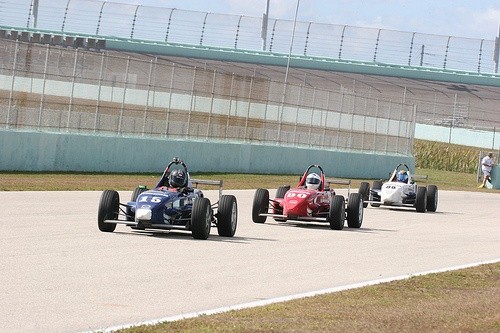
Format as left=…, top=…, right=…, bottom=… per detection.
left=397, top=170, right=408, bottom=183
left=298, top=173, right=321, bottom=190
left=478, top=152, right=494, bottom=187
left=159, top=168, right=188, bottom=194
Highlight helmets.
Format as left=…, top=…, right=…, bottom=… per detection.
left=169, top=169, right=187, bottom=187
left=305, top=173, right=321, bottom=189
left=397, top=170, right=408, bottom=182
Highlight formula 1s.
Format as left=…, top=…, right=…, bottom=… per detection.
left=252, top=178, right=365, bottom=230
left=358, top=177, right=438, bottom=213
left=97, top=179, right=238, bottom=240
